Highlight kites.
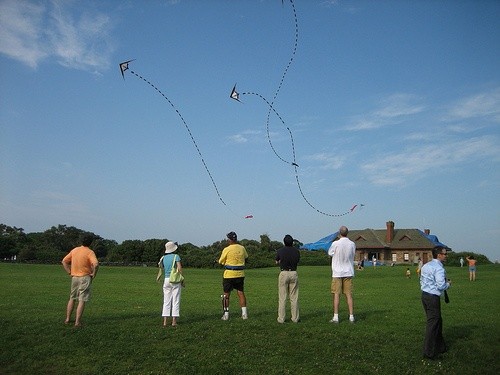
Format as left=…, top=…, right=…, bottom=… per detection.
left=119, top=58, right=137, bottom=81
left=229, top=81, right=245, bottom=104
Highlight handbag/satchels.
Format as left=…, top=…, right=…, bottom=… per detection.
left=169, top=254, right=185, bottom=284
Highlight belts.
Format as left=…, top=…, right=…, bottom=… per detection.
left=280, top=268, right=297, bottom=272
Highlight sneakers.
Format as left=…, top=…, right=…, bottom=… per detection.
left=349, top=316, right=355, bottom=323
left=329, top=319, right=339, bottom=324
left=241, top=314, right=248, bottom=320
left=221, top=315, right=229, bottom=321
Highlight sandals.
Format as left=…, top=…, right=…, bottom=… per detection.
left=74, top=322, right=82, bottom=328
left=172, top=323, right=178, bottom=326
left=64, top=319, right=71, bottom=325
left=163, top=324, right=166, bottom=327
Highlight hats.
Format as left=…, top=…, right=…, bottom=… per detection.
left=164, top=241, right=178, bottom=253
left=226, top=232, right=237, bottom=240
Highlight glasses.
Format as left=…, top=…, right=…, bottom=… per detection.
left=440, top=252, right=448, bottom=255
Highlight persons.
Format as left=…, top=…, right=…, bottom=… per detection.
left=405, top=268, right=411, bottom=279
left=62, top=232, right=99, bottom=328
left=214, top=232, right=249, bottom=320
left=466, top=255, right=478, bottom=280
left=157, top=241, right=186, bottom=326
left=357, top=258, right=364, bottom=270
left=420, top=246, right=453, bottom=360
left=327, top=226, right=356, bottom=324
left=458, top=256, right=467, bottom=267
left=276, top=234, right=300, bottom=323
left=372, top=255, right=377, bottom=270
left=416, top=258, right=423, bottom=282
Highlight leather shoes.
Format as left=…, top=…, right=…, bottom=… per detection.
left=438, top=345, right=450, bottom=353
left=422, top=355, right=441, bottom=362
